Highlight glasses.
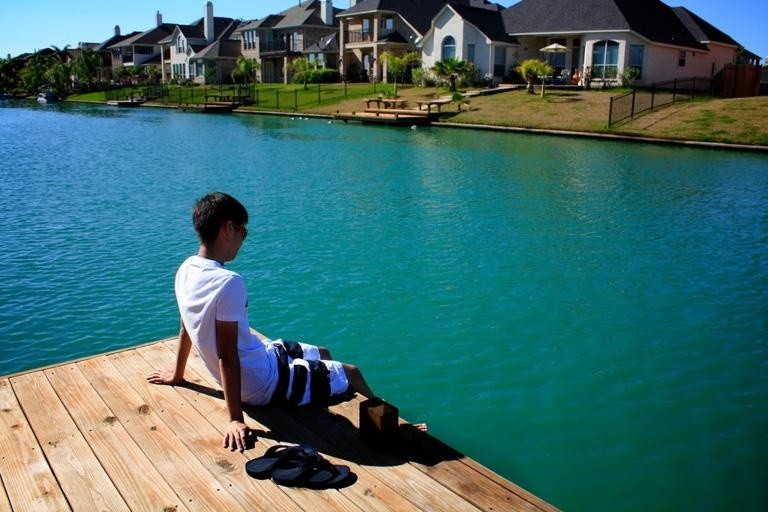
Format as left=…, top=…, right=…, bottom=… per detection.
left=231, top=220, right=249, bottom=239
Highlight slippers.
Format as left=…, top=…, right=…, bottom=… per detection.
left=272, top=453, right=352, bottom=489
left=243, top=442, right=319, bottom=480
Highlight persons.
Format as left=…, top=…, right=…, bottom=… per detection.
left=145, top=194, right=428, bottom=454
left=560, top=64, right=569, bottom=85
left=572, top=66, right=579, bottom=84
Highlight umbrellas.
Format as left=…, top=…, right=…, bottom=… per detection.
left=539, top=43, right=570, bottom=78
